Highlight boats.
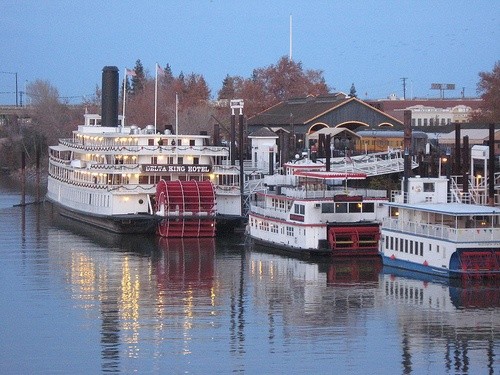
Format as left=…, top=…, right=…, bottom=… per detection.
left=242, top=107, right=500, bottom=279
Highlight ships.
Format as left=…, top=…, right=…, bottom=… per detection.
left=44, top=66, right=246, bottom=239
